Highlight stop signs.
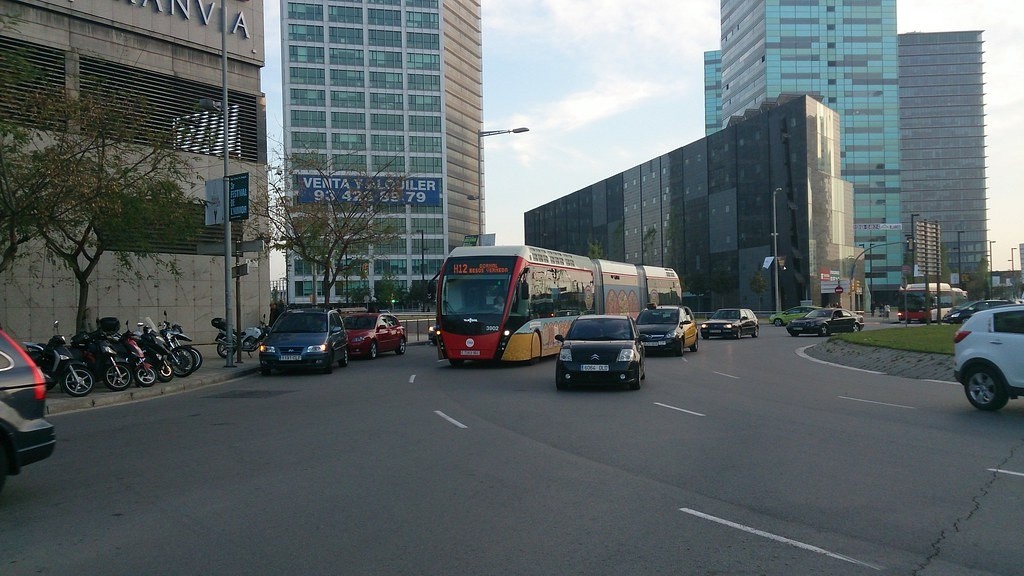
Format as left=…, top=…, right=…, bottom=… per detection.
left=835, top=287, right=843, bottom=294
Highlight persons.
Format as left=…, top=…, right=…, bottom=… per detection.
left=668, top=312, right=678, bottom=323
left=825, top=301, right=842, bottom=309
left=269, top=299, right=287, bottom=327
left=614, top=322, right=630, bottom=339
left=493, top=292, right=505, bottom=312
left=884, top=302, right=890, bottom=318
left=879, top=303, right=885, bottom=318
left=870, top=301, right=876, bottom=317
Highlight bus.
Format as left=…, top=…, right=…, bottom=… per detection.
left=428, top=245, right=683, bottom=367
left=898, top=283, right=969, bottom=324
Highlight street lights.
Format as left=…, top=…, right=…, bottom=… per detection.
left=953, top=231, right=965, bottom=288
left=911, top=213, right=920, bottom=284
left=773, top=187, right=782, bottom=312
left=870, top=244, right=875, bottom=316
left=467, top=128, right=530, bottom=246
left=986, top=241, right=996, bottom=299
left=1008, top=247, right=1017, bottom=299
left=416, top=229, right=425, bottom=313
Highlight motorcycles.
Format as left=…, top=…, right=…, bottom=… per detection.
left=23, top=310, right=203, bottom=398
left=211, top=314, right=272, bottom=359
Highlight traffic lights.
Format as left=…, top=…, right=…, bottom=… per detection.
left=391, top=299, right=396, bottom=303
left=850, top=279, right=860, bottom=292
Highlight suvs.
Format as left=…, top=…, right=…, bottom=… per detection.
left=953, top=304, right=1024, bottom=411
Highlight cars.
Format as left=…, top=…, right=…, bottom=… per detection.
left=700, top=309, right=759, bottom=340
left=943, top=299, right=1024, bottom=324
left=555, top=315, right=645, bottom=391
left=259, top=307, right=406, bottom=376
left=428, top=325, right=438, bottom=344
left=786, top=308, right=864, bottom=337
left=0, top=330, right=57, bottom=493
left=769, top=306, right=822, bottom=327
left=635, top=302, right=699, bottom=356
